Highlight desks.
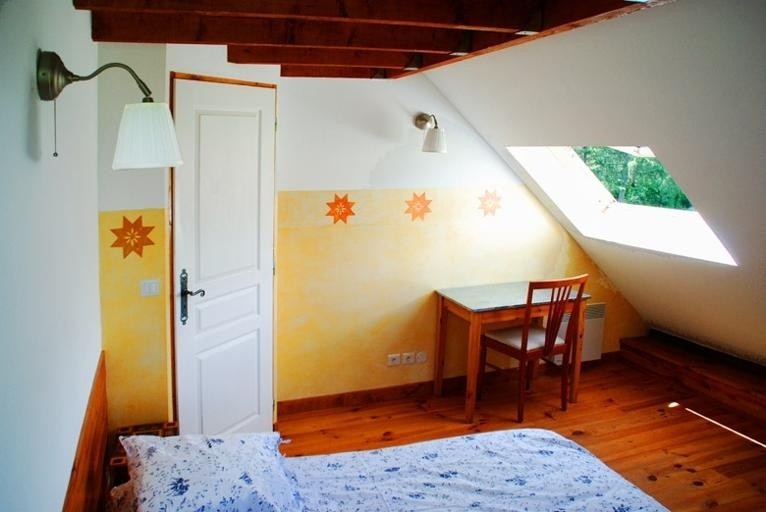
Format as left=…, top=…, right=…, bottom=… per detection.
left=434, top=281, right=591, bottom=424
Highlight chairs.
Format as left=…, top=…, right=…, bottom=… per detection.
left=476, top=272, right=591, bottom=423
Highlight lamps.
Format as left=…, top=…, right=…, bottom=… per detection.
left=34, top=47, right=185, bottom=170
left=414, top=112, right=446, bottom=153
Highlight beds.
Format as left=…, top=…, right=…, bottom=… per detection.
left=110, top=425, right=670, bottom=512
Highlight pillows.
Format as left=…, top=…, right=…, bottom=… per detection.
left=117, top=430, right=297, bottom=512
